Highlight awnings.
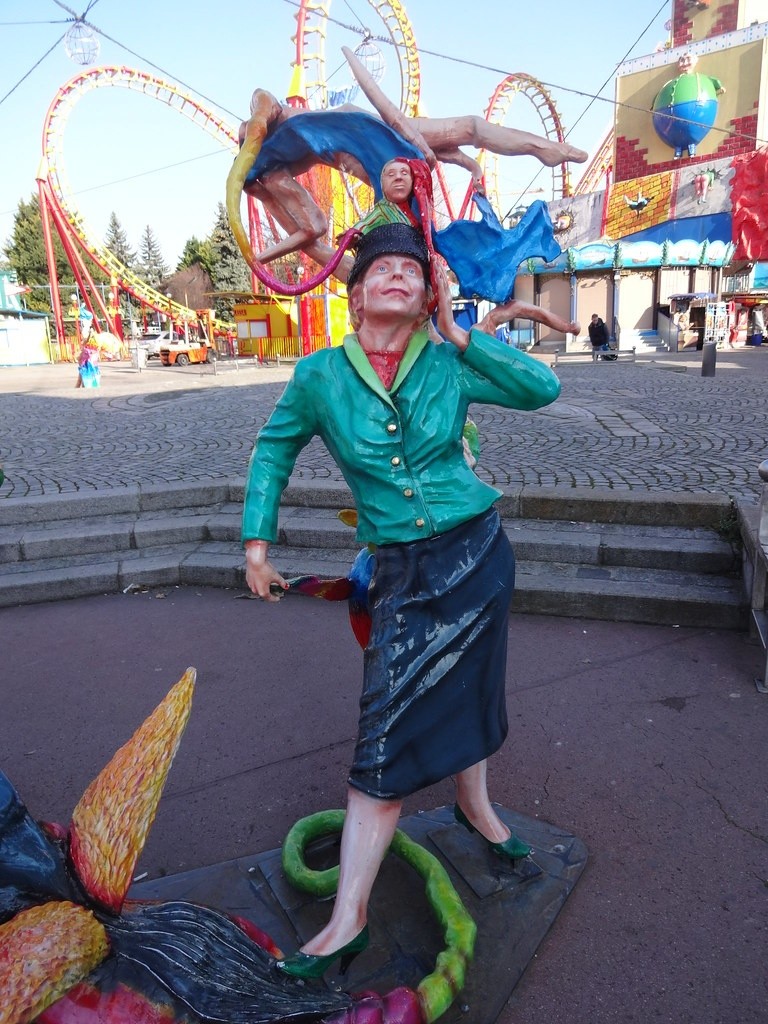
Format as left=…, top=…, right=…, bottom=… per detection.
left=669, top=293, right=715, bottom=302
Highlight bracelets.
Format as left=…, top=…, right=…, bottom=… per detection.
left=244, top=539, right=269, bottom=548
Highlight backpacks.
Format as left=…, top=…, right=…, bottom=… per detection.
left=601, top=345, right=618, bottom=361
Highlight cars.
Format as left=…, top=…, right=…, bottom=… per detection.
left=129, top=331, right=180, bottom=359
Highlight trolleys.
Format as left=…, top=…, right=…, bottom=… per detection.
left=514, top=318, right=532, bottom=351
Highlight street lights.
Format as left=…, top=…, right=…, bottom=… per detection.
left=140, top=293, right=149, bottom=333
left=108, top=292, right=117, bottom=336
left=166, top=293, right=173, bottom=320
left=72, top=293, right=83, bottom=350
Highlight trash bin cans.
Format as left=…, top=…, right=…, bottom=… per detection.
left=752, top=333, right=762, bottom=347
left=701, top=342, right=717, bottom=377
left=129, top=344, right=149, bottom=368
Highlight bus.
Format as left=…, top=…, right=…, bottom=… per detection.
left=137, top=327, right=161, bottom=334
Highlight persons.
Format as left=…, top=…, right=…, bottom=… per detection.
left=588, top=313, right=609, bottom=361
left=650, top=50, right=726, bottom=160
left=234, top=46, right=585, bottom=338
left=240, top=222, right=567, bottom=985
left=73, top=329, right=99, bottom=389
left=694, top=171, right=715, bottom=205
